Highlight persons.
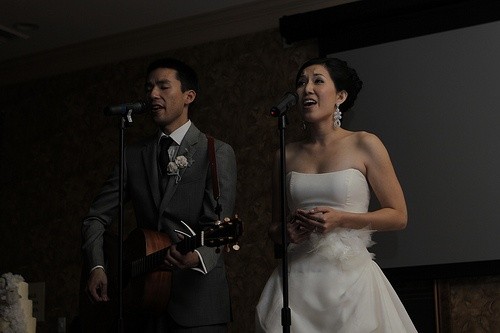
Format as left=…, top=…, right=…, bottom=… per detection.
left=82, top=58, right=238, bottom=333
left=255, top=58, right=418, bottom=333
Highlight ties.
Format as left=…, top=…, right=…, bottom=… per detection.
left=158, top=136, right=175, bottom=199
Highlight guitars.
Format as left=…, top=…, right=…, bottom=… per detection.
left=79, top=214, right=244, bottom=320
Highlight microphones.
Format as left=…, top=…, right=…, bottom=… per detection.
left=270, top=91, right=298, bottom=117
left=104, top=99, right=149, bottom=117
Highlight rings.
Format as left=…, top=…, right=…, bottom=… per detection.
left=312, top=227, right=317, bottom=233
left=170, top=264, right=173, bottom=268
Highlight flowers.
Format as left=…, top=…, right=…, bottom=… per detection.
left=167, top=156, right=189, bottom=184
